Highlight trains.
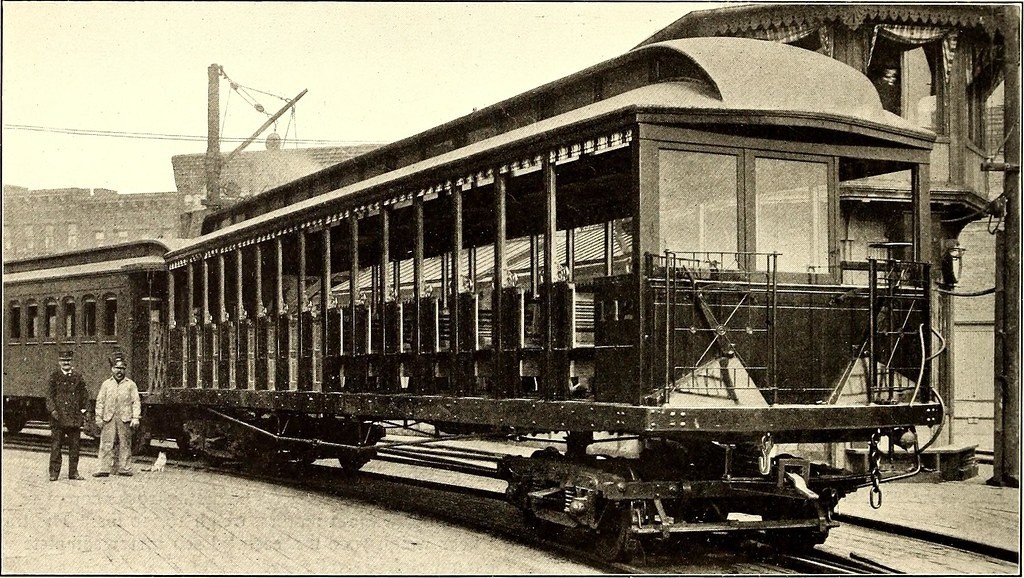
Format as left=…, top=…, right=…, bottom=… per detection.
left=3, top=37, right=947, bottom=562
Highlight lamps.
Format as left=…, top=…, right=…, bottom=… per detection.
left=948, top=241, right=965, bottom=261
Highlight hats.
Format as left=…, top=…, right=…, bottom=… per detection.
left=58, top=351, right=73, bottom=360
left=109, top=352, right=126, bottom=366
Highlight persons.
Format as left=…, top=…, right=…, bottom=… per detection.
left=47, top=351, right=89, bottom=480
left=93, top=357, right=141, bottom=477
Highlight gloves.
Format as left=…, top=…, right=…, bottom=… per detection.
left=129, top=419, right=139, bottom=430
left=52, top=410, right=59, bottom=420
left=96, top=419, right=104, bottom=429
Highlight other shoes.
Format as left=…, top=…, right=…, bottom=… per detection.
left=118, top=470, right=134, bottom=476
left=92, top=472, right=110, bottom=477
left=69, top=475, right=83, bottom=480
left=50, top=476, right=58, bottom=481
